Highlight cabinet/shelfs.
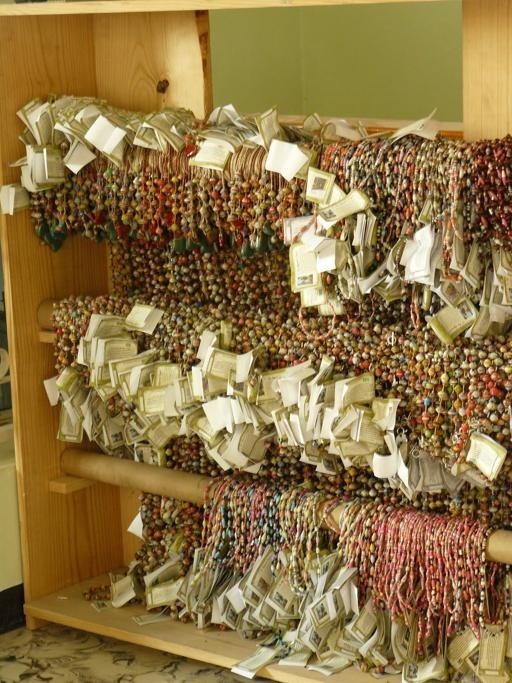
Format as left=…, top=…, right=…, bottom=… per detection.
left=0, top=1, right=512, bottom=678
